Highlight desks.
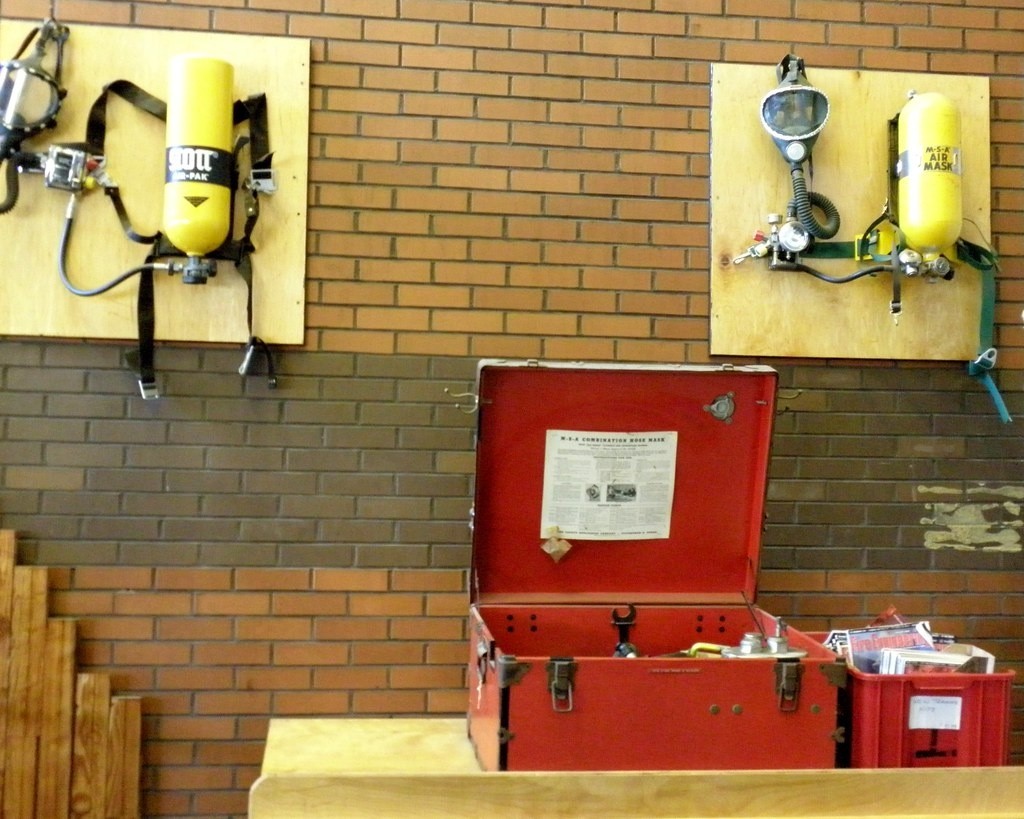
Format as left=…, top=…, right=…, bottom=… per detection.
left=249, top=717, right=1024, bottom=819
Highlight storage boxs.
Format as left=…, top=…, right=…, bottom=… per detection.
left=443, top=354, right=844, bottom=773
left=804, top=631, right=1018, bottom=769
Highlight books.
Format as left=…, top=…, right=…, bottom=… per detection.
left=832, top=620, right=973, bottom=674
